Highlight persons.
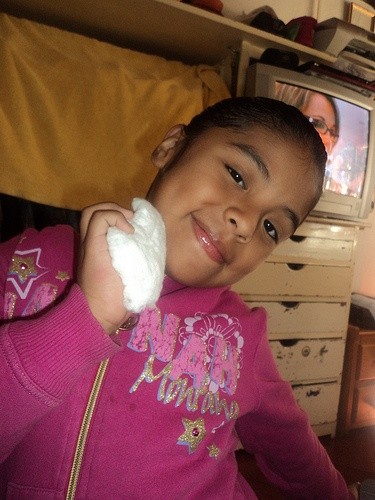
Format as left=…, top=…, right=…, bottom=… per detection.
left=0, top=94, right=361, bottom=500
left=276, top=81, right=341, bottom=158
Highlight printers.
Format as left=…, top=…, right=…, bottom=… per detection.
left=314, top=2, right=375, bottom=69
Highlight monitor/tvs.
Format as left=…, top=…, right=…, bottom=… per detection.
left=246, top=62, right=375, bottom=223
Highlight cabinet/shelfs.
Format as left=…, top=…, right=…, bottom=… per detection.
left=341, top=330, right=375, bottom=432
left=230, top=221, right=360, bottom=451
